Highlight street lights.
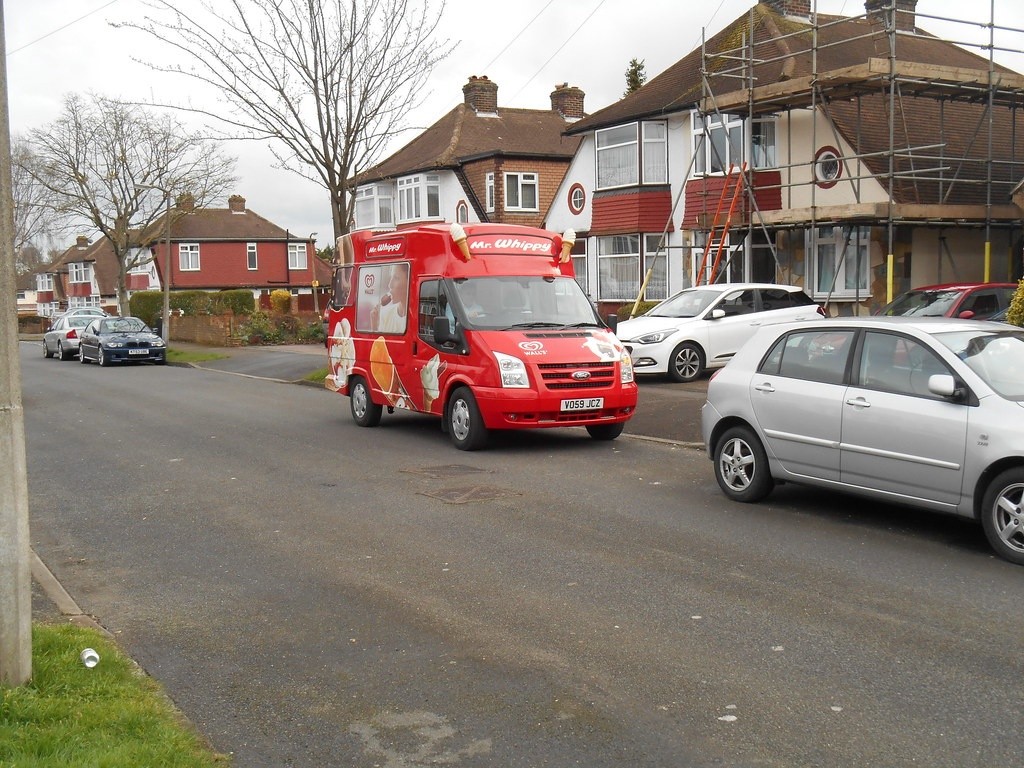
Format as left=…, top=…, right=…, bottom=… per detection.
left=134, top=184, right=171, bottom=353
left=310, top=233, right=319, bottom=313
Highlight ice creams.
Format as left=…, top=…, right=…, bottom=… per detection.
left=449, top=221, right=471, bottom=260
left=329, top=318, right=447, bottom=412
left=561, top=226, right=576, bottom=262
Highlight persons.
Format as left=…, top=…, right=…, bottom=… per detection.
left=445, top=282, right=484, bottom=334
left=371, top=264, right=408, bottom=333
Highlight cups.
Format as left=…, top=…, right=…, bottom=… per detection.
left=382, top=365, right=418, bottom=412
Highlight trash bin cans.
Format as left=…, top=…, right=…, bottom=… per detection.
left=150, top=316, right=162, bottom=338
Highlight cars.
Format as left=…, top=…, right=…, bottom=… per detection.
left=42, top=315, right=104, bottom=360
left=807, top=282, right=1021, bottom=366
left=47, top=307, right=112, bottom=331
left=78, top=317, right=167, bottom=367
left=322, top=296, right=347, bottom=347
left=702, top=315, right=1024, bottom=564
left=616, top=283, right=827, bottom=383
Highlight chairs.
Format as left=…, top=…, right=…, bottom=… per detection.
left=501, top=289, right=534, bottom=325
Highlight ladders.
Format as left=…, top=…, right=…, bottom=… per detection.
left=695, top=161, right=748, bottom=287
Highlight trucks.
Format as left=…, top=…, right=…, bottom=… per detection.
left=325, top=221, right=638, bottom=451
